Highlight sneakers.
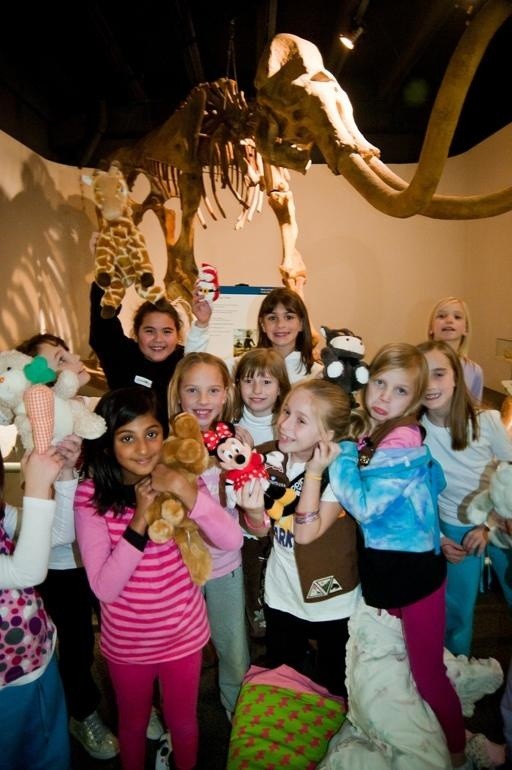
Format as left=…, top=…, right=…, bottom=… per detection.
left=69, top=711, right=120, bottom=759
left=146, top=705, right=164, bottom=740
left=155, top=733, right=173, bottom=770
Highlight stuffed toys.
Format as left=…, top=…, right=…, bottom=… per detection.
left=196, top=264, right=219, bottom=302
left=90, top=161, right=171, bottom=319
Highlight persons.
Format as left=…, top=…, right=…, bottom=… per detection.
left=2, top=285, right=511, bottom=767
